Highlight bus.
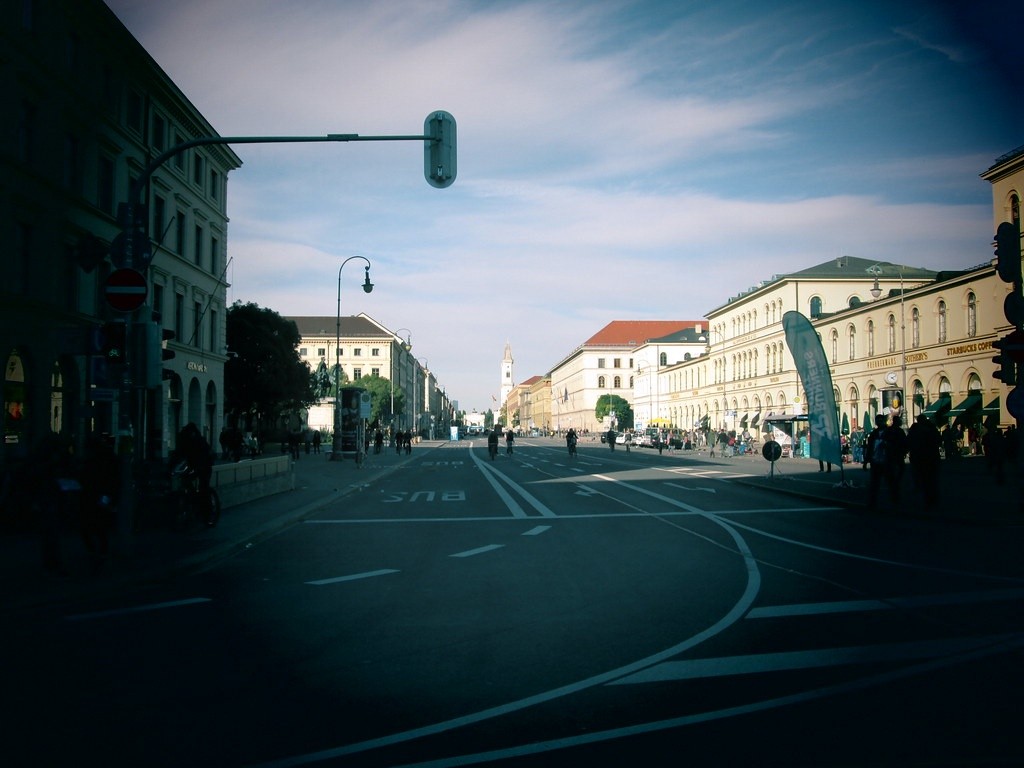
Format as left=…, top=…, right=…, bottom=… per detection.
left=636, top=427, right=684, bottom=449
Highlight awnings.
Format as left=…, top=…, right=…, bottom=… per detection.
left=646, top=418, right=672, bottom=423
left=734, top=411, right=772, bottom=425
left=921, top=393, right=1000, bottom=416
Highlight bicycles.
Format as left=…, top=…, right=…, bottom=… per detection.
left=488, top=442, right=497, bottom=461
left=507, top=440, right=514, bottom=458
left=165, top=474, right=222, bottom=533
left=607, top=437, right=615, bottom=452
left=625, top=439, right=632, bottom=453
left=396, top=439, right=409, bottom=456
left=568, top=437, right=578, bottom=458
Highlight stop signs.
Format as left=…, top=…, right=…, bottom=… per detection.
left=103, top=268, right=148, bottom=313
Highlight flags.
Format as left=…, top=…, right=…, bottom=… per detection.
left=782, top=310, right=843, bottom=468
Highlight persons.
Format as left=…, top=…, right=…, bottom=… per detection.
left=863, top=415, right=1024, bottom=510
left=396, top=429, right=412, bottom=455
left=488, top=431, right=498, bottom=456
left=220, top=425, right=267, bottom=463
left=608, top=428, right=616, bottom=448
left=797, top=426, right=865, bottom=465
left=287, top=428, right=321, bottom=460
left=30, top=423, right=213, bottom=576
left=365, top=430, right=383, bottom=454
left=624, top=431, right=631, bottom=452
left=654, top=428, right=750, bottom=457
left=566, top=428, right=578, bottom=451
left=506, top=430, right=514, bottom=454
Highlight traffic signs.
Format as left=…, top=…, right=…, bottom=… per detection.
left=104, top=322, right=126, bottom=364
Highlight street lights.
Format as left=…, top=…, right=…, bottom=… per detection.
left=391, top=327, right=412, bottom=447
left=868, top=261, right=909, bottom=436
left=637, top=359, right=653, bottom=428
left=332, top=256, right=374, bottom=461
left=600, top=374, right=613, bottom=421
left=413, top=357, right=429, bottom=438
left=703, top=330, right=727, bottom=436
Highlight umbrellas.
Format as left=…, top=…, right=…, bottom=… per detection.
left=864, top=411, right=873, bottom=437
left=841, top=412, right=850, bottom=441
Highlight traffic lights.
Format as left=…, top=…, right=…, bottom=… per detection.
left=131, top=321, right=176, bottom=387
left=992, top=336, right=1015, bottom=386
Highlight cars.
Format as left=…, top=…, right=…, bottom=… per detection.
left=601, top=432, right=639, bottom=446
